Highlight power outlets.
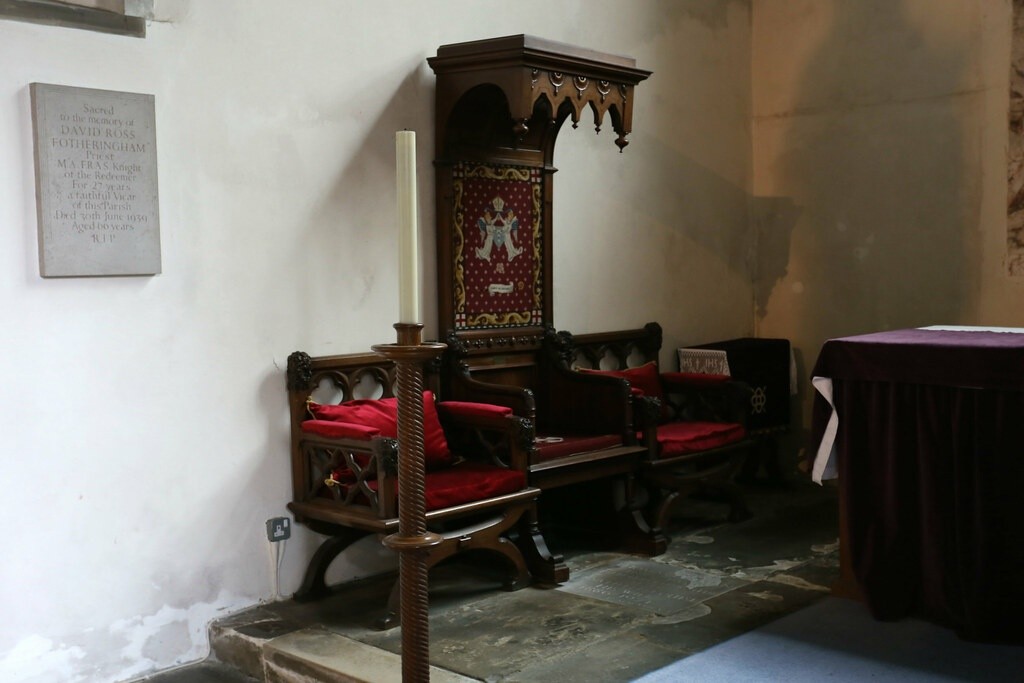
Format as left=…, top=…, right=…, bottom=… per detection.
left=268, top=516, right=291, bottom=543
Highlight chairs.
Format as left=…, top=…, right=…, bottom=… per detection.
left=283, top=341, right=543, bottom=633
left=557, top=320, right=758, bottom=525
left=425, top=33, right=670, bottom=584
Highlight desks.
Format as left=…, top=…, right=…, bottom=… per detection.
left=805, top=321, right=1024, bottom=647
left=676, top=334, right=794, bottom=490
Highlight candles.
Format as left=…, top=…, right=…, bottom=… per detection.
left=395, top=127, right=422, bottom=325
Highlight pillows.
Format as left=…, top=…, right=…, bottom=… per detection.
left=574, top=359, right=680, bottom=429
left=305, top=388, right=456, bottom=476
